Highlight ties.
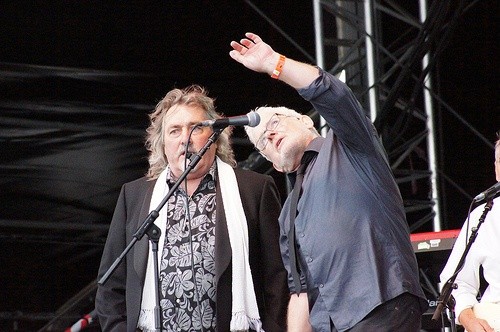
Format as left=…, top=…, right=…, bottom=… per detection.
left=288, top=151, right=317, bottom=297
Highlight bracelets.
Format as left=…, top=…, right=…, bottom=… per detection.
left=271, top=55, right=285, bottom=80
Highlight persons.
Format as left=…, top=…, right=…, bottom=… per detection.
left=94, top=84, right=284, bottom=332
left=229, top=33, right=430, bottom=332
left=439, top=138, right=500, bottom=332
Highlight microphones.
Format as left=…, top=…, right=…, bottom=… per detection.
left=473, top=181, right=500, bottom=203
left=198, top=111, right=260, bottom=127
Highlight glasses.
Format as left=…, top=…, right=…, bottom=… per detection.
left=256, top=113, right=299, bottom=159
